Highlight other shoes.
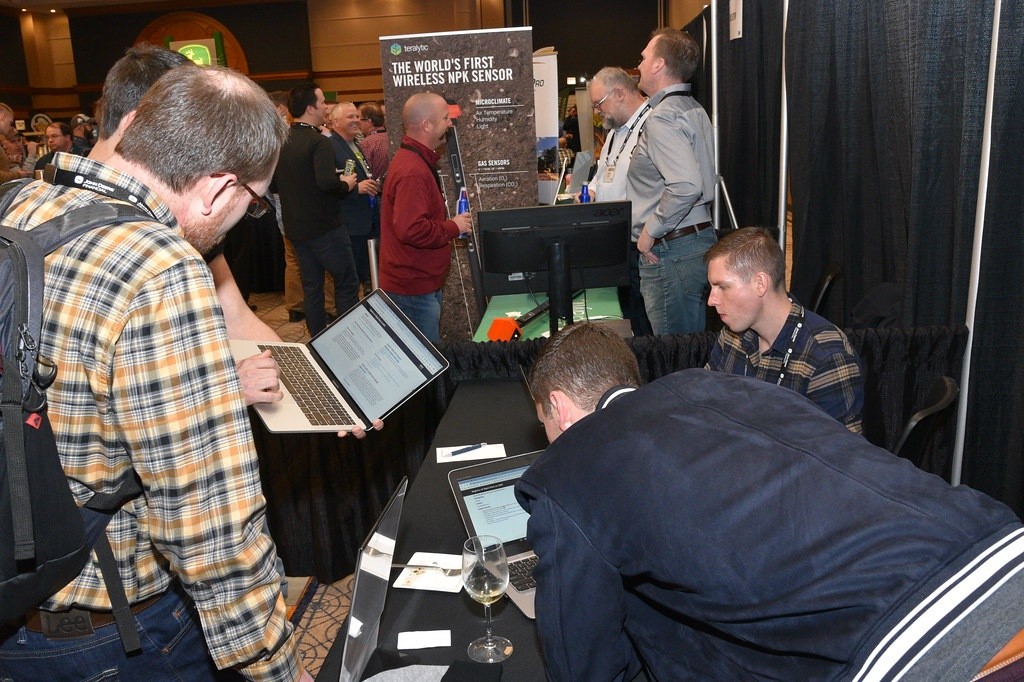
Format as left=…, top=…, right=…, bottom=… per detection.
left=325, top=313, right=338, bottom=323
left=288, top=309, right=307, bottom=323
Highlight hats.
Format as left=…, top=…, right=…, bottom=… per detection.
left=70, top=113, right=97, bottom=129
left=1, top=126, right=24, bottom=140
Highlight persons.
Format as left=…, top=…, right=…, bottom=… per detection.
left=0, top=103, right=31, bottom=181
left=573, top=65, right=654, bottom=336
left=1, top=126, right=36, bottom=178
left=0, top=41, right=313, bottom=682
left=220, top=83, right=388, bottom=337
left=380, top=94, right=472, bottom=341
left=34, top=122, right=85, bottom=177
left=702, top=226, right=864, bottom=435
left=626, top=26, right=715, bottom=336
left=70, top=112, right=96, bottom=156
left=513, top=322, right=1024, bottom=682
left=558, top=105, right=581, bottom=171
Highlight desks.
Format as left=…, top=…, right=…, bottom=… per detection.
left=315, top=380, right=557, bottom=682
left=471, top=289, right=633, bottom=344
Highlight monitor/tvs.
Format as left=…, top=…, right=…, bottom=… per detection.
left=478, top=200, right=632, bottom=338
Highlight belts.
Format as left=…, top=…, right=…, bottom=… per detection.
left=654, top=221, right=712, bottom=246
left=24, top=588, right=168, bottom=635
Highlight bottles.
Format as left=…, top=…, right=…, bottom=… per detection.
left=367, top=173, right=376, bottom=208
left=579, top=181, right=590, bottom=203
left=457, top=191, right=469, bottom=239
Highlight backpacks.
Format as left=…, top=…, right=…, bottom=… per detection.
left=0, top=177, right=163, bottom=653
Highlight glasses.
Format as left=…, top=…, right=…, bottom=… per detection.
left=46, top=134, right=64, bottom=139
left=591, top=92, right=613, bottom=110
left=209, top=172, right=272, bottom=219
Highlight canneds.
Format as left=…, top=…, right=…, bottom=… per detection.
left=344, top=159, right=356, bottom=176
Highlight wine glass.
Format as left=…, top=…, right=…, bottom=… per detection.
left=461, top=534, right=513, bottom=663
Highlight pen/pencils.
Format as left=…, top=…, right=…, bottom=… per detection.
left=443, top=442, right=487, bottom=458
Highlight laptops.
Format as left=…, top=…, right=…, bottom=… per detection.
left=448, top=449, right=547, bottom=620
left=227, top=289, right=449, bottom=432
